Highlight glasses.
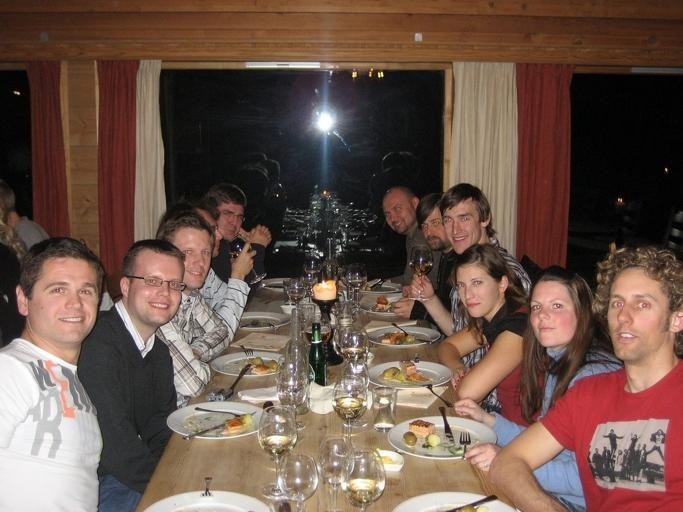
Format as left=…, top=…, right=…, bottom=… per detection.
left=125, top=274, right=187, bottom=291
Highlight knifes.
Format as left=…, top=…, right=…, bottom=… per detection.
left=228, top=365, right=250, bottom=395
left=438, top=404, right=455, bottom=447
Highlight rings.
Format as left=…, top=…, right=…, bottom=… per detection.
left=482, top=461, right=487, bottom=467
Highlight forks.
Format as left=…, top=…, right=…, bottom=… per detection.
left=423, top=381, right=451, bottom=409
left=199, top=474, right=213, bottom=498
left=459, top=430, right=471, bottom=459
left=237, top=340, right=254, bottom=354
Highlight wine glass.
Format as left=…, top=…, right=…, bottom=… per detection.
left=228, top=235, right=267, bottom=284
left=409, top=242, right=435, bottom=301
left=273, top=183, right=287, bottom=201
left=258, top=257, right=385, bottom=507
left=298, top=190, right=348, bottom=256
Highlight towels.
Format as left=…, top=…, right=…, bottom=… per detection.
left=380, top=385, right=450, bottom=409
left=362, top=319, right=417, bottom=332
left=308, top=380, right=372, bottom=414
left=229, top=331, right=292, bottom=352
left=238, top=386, right=279, bottom=403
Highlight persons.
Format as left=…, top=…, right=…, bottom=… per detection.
left=487, top=247, right=682, bottom=512
left=204, top=182, right=272, bottom=307
left=169, top=200, right=256, bottom=342
left=437, top=242, right=551, bottom=429
left=0, top=175, right=53, bottom=350
left=451, top=268, right=624, bottom=512
left=388, top=190, right=464, bottom=327
left=74, top=238, right=186, bottom=511
left=412, top=183, right=533, bottom=414
left=0, top=235, right=107, bottom=512
left=153, top=209, right=230, bottom=408
left=382, top=186, right=442, bottom=291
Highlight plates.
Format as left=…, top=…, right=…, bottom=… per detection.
left=259, top=277, right=292, bottom=290
left=387, top=492, right=515, bottom=511
left=207, top=351, right=283, bottom=378
left=367, top=324, right=442, bottom=352
left=361, top=294, right=406, bottom=317
left=144, top=491, right=270, bottom=512
left=392, top=411, right=497, bottom=463
left=168, top=400, right=269, bottom=441
left=358, top=277, right=403, bottom=295
left=364, top=359, right=453, bottom=392
left=234, top=308, right=290, bottom=333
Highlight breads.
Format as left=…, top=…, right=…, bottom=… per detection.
left=224, top=418, right=242, bottom=433
left=379, top=332, right=398, bottom=344
left=409, top=419, right=435, bottom=438
left=400, top=359, right=416, bottom=375
left=251, top=364, right=271, bottom=375
